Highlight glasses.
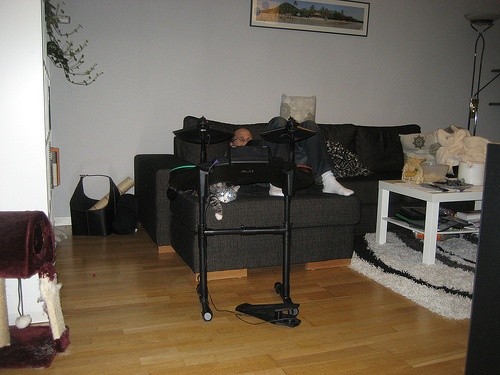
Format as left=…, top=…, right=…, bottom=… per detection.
left=233, top=137, right=252, bottom=143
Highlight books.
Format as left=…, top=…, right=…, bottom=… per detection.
left=50, top=147, right=60, bottom=188
left=454, top=209, right=481, bottom=221
left=394, top=206, right=454, bottom=228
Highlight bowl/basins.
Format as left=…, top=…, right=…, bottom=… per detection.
left=457, top=161, right=486, bottom=185
left=420, top=163, right=449, bottom=182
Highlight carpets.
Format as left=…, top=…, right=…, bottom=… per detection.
left=353, top=214, right=485, bottom=320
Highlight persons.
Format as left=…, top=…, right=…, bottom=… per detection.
left=212, top=117, right=354, bottom=196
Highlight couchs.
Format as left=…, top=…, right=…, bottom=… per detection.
left=134, top=154, right=475, bottom=274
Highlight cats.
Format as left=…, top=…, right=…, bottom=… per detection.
left=166, top=181, right=241, bottom=221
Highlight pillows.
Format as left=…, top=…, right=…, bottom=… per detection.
left=174, top=94, right=468, bottom=200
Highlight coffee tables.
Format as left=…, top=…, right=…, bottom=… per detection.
left=377, top=179, right=486, bottom=267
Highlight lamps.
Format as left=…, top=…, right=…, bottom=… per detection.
left=462, top=12, right=500, bottom=138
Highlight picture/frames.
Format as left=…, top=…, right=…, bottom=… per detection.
left=250, top=0, right=370, bottom=36
